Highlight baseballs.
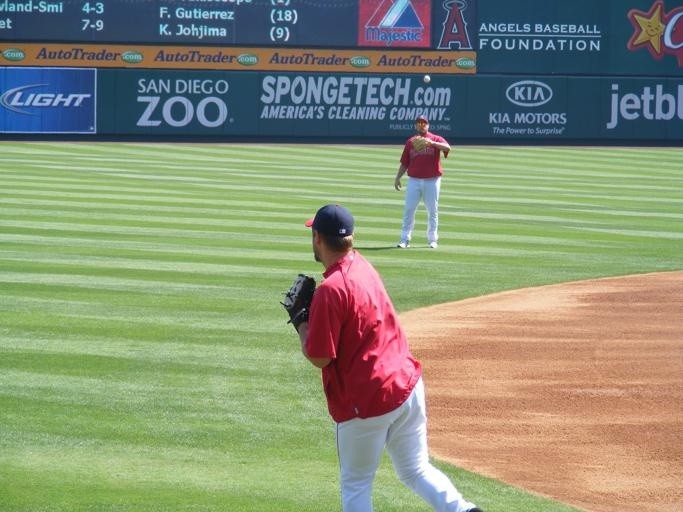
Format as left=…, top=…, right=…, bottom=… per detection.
left=424, top=75, right=431, bottom=83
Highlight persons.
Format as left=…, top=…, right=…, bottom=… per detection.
left=283, top=201, right=481, bottom=512
left=393, top=113, right=452, bottom=249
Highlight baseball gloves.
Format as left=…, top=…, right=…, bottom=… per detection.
left=412, top=136, right=431, bottom=151
left=285, top=273, right=315, bottom=325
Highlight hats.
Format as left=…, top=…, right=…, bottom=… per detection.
left=415, top=115, right=429, bottom=123
left=305, top=204, right=355, bottom=237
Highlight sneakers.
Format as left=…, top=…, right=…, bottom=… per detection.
left=396, top=239, right=410, bottom=248
left=428, top=241, right=438, bottom=248
